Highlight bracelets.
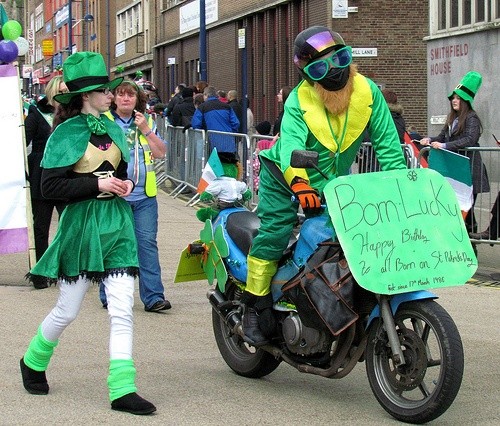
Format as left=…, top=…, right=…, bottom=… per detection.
left=144, top=130, right=152, bottom=137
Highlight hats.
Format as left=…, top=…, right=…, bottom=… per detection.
left=447, top=71, right=482, bottom=112
left=182, top=88, right=193, bottom=97
left=255, top=121, right=271, bottom=135
left=53, top=53, right=124, bottom=105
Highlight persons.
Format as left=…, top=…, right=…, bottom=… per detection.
left=19, top=52, right=190, bottom=414
left=241, top=26, right=411, bottom=345
left=19, top=71, right=500, bottom=312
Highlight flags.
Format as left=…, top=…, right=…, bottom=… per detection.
left=427, top=143, right=473, bottom=220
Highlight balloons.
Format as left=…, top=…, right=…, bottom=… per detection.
left=0, top=20, right=29, bottom=63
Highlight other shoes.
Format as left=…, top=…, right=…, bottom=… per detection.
left=19, top=358, right=49, bottom=394
left=244, top=305, right=265, bottom=342
left=470, top=228, right=495, bottom=247
left=112, top=393, right=156, bottom=415
left=150, top=300, right=171, bottom=312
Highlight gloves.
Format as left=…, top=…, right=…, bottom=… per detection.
left=182, top=124, right=192, bottom=133
left=290, top=182, right=321, bottom=216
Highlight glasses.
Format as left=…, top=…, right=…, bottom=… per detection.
left=59, top=88, right=69, bottom=93
left=304, top=46, right=352, bottom=81
left=94, top=89, right=109, bottom=95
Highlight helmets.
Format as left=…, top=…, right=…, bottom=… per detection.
left=293, top=25, right=346, bottom=86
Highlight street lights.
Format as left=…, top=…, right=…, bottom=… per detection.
left=68, top=14, right=94, bottom=56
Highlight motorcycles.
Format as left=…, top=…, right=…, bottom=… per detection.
left=187, top=149, right=465, bottom=425
